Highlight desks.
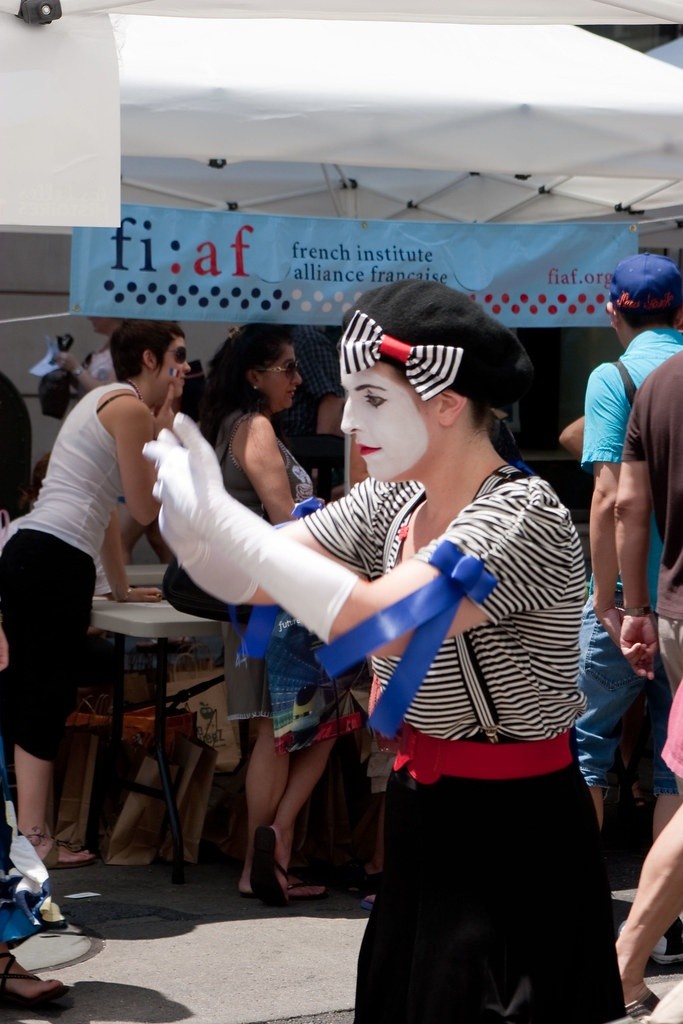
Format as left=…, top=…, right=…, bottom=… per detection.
left=91, top=599, right=230, bottom=884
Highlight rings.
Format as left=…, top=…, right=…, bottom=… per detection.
left=148, top=411, right=155, bottom=417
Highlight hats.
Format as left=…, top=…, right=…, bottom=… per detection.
left=609, top=252, right=683, bottom=313
left=336, top=278, right=535, bottom=409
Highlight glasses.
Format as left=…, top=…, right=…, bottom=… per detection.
left=163, top=346, right=187, bottom=364
left=251, top=360, right=302, bottom=379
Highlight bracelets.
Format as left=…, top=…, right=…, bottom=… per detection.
left=119, top=587, right=133, bottom=602
left=625, top=603, right=653, bottom=616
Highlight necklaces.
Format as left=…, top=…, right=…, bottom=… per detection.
left=125, top=377, right=145, bottom=402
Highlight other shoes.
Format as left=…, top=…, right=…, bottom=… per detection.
left=625, top=986, right=661, bottom=1023
left=616, top=915, right=683, bottom=964
left=331, top=854, right=384, bottom=897
left=359, top=894, right=376, bottom=910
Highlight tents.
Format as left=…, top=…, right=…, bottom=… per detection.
left=0, top=0, right=683, bottom=494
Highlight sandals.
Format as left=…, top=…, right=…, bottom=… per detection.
left=41, top=839, right=95, bottom=871
left=0, top=951, right=70, bottom=1007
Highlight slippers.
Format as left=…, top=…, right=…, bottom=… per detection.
left=240, top=882, right=330, bottom=900
left=250, top=824, right=292, bottom=907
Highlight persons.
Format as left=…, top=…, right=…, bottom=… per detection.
left=0, top=250, right=683, bottom=1024
left=142, top=278, right=626, bottom=1024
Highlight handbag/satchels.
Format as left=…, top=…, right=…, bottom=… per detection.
left=163, top=556, right=251, bottom=625
left=39, top=333, right=74, bottom=419
left=53, top=641, right=244, bottom=866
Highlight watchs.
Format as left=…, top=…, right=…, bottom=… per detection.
left=72, top=367, right=84, bottom=376
